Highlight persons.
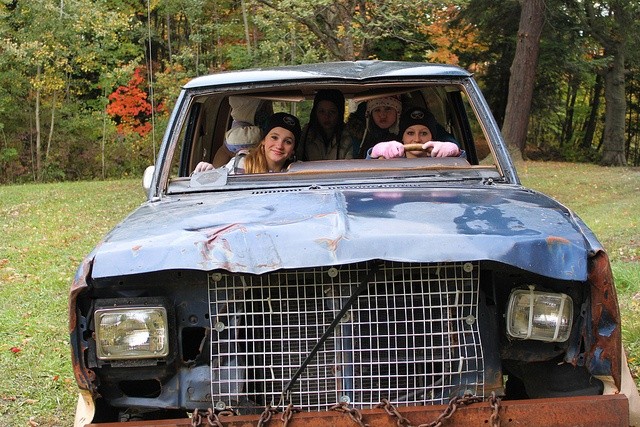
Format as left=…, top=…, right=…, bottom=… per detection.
left=296, top=89, right=353, bottom=160
left=346, top=97, right=461, bottom=148
left=193, top=112, right=303, bottom=175
left=366, top=98, right=469, bottom=160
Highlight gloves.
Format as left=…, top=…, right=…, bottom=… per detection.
left=371, top=140, right=405, bottom=159
left=422, top=141, right=460, bottom=157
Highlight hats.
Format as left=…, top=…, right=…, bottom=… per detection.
left=261, top=111, right=301, bottom=150
left=398, top=107, right=438, bottom=140
left=360, top=94, right=402, bottom=147
left=304, top=88, right=345, bottom=159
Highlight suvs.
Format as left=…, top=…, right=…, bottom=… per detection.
left=70, top=60, right=639, bottom=427
